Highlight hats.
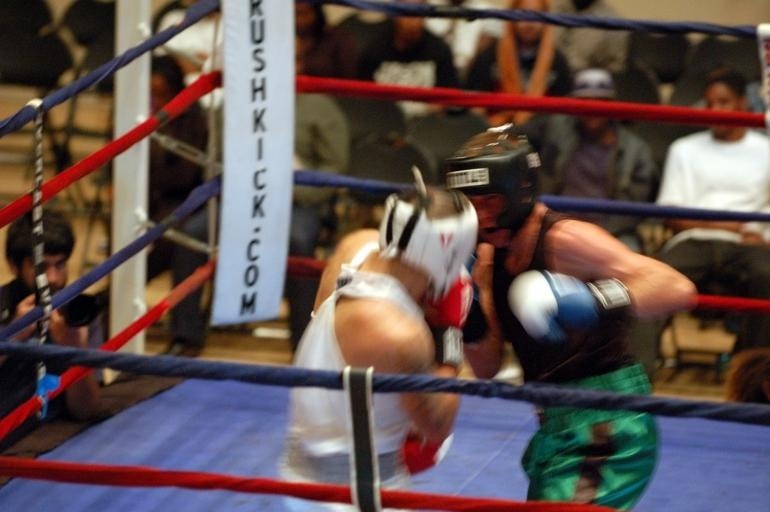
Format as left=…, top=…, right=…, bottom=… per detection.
left=569, top=68, right=618, bottom=99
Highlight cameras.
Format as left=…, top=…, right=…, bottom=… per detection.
left=48, top=282, right=99, bottom=327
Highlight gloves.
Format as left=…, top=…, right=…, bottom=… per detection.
left=504, top=270, right=634, bottom=346
left=421, top=264, right=474, bottom=366
left=400, top=428, right=454, bottom=473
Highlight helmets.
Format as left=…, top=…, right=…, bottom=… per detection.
left=378, top=122, right=542, bottom=303
